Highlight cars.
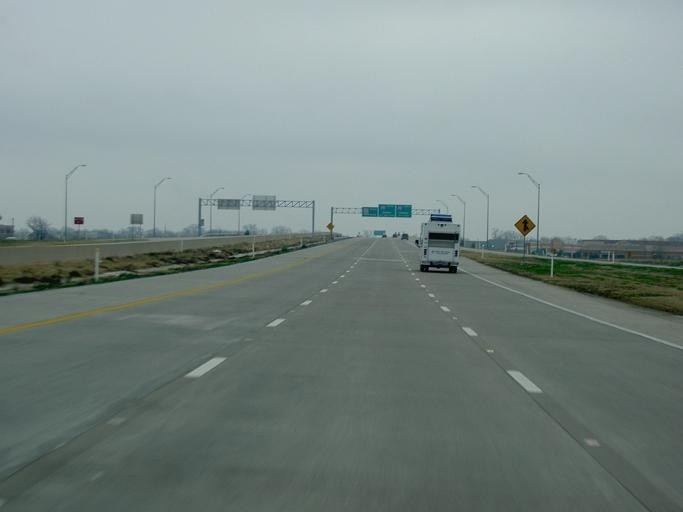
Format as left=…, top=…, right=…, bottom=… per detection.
left=401, top=234, right=409, bottom=240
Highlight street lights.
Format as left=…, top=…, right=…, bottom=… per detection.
left=472, top=186, right=489, bottom=242
left=237, top=193, right=251, bottom=231
left=209, top=187, right=224, bottom=232
left=518, top=172, right=540, bottom=250
left=436, top=199, right=448, bottom=213
left=154, top=177, right=171, bottom=234
left=450, top=194, right=465, bottom=246
left=64, top=163, right=86, bottom=240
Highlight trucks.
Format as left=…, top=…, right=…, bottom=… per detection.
left=415, top=214, right=459, bottom=272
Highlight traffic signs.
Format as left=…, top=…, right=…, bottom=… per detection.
left=362, top=204, right=411, bottom=217
